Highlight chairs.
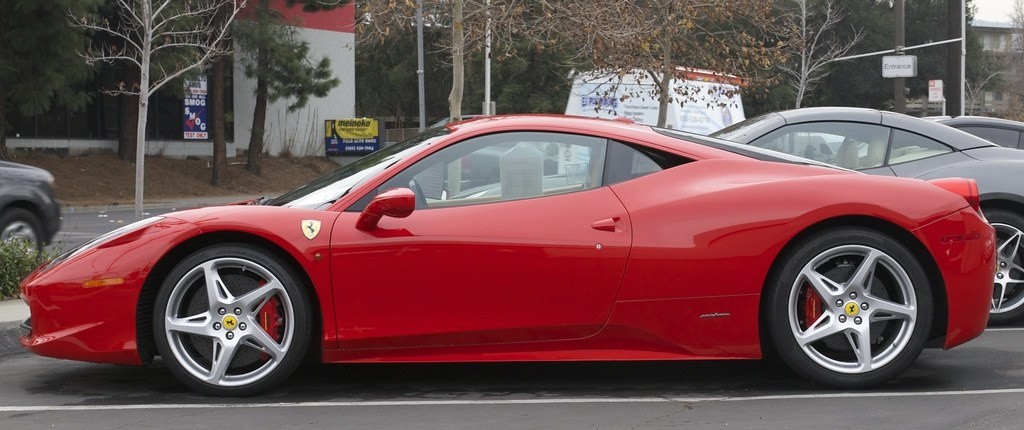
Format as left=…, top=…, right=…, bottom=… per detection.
left=835, top=140, right=859, bottom=168
left=866, top=131, right=894, bottom=167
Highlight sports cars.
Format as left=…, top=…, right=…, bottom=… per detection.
left=706, top=107, right=1024, bottom=331
left=15, top=117, right=995, bottom=400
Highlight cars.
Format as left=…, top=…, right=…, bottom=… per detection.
left=936, top=116, right=1024, bottom=154
left=0, top=160, right=67, bottom=266
